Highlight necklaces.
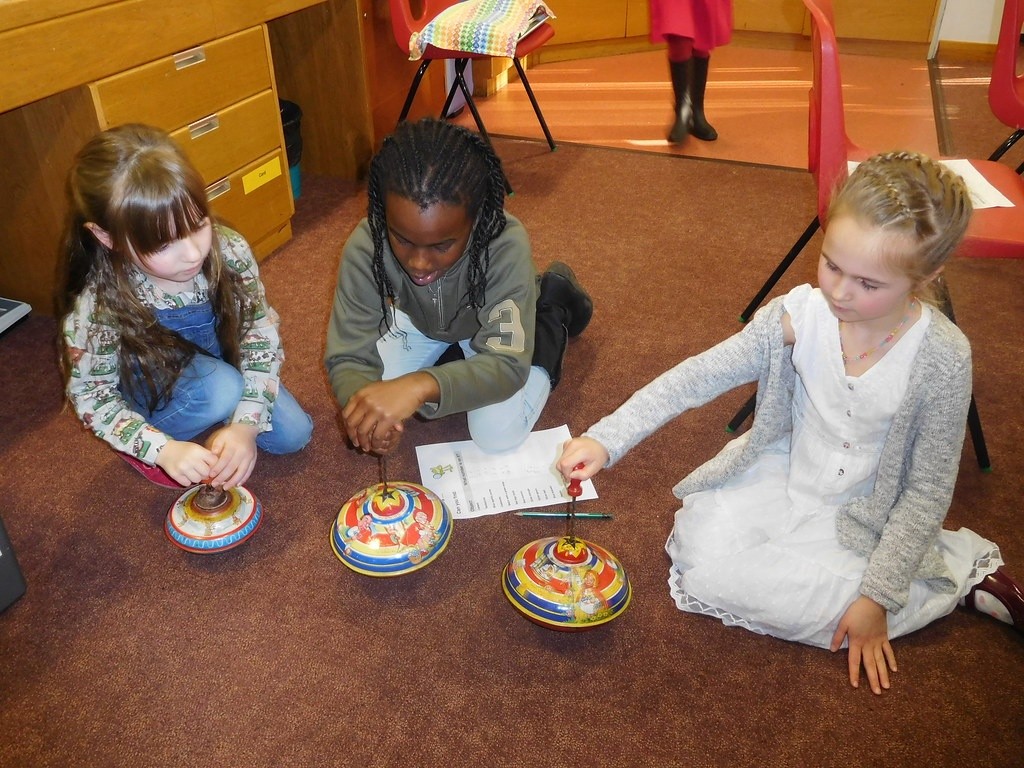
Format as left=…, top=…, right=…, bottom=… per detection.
left=838, top=297, right=915, bottom=365
left=427, top=269, right=449, bottom=307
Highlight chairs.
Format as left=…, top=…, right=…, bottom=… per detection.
left=986, top=0, right=1024, bottom=177
left=388, top=0, right=556, bottom=197
left=727, top=0, right=1024, bottom=478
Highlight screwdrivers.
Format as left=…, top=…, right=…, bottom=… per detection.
left=568, top=462, right=585, bottom=547
left=382, top=455, right=388, bottom=496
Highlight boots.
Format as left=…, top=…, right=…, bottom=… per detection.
left=667, top=52, right=718, bottom=143
left=531, top=262, right=593, bottom=393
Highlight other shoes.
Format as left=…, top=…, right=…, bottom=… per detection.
left=113, top=449, right=199, bottom=492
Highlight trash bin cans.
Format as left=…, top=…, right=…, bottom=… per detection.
left=278, top=97, right=303, bottom=200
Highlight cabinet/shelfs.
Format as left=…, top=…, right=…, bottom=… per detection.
left=0, top=0, right=444, bottom=302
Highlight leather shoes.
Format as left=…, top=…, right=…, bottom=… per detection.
left=956, top=569, right=1023, bottom=633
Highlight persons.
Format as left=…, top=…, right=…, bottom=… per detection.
left=646, top=1, right=735, bottom=144
left=325, top=117, right=593, bottom=459
left=55, top=123, right=314, bottom=492
left=555, top=152, right=1024, bottom=693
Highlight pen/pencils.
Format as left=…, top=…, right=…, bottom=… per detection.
left=513, top=511, right=613, bottom=519
